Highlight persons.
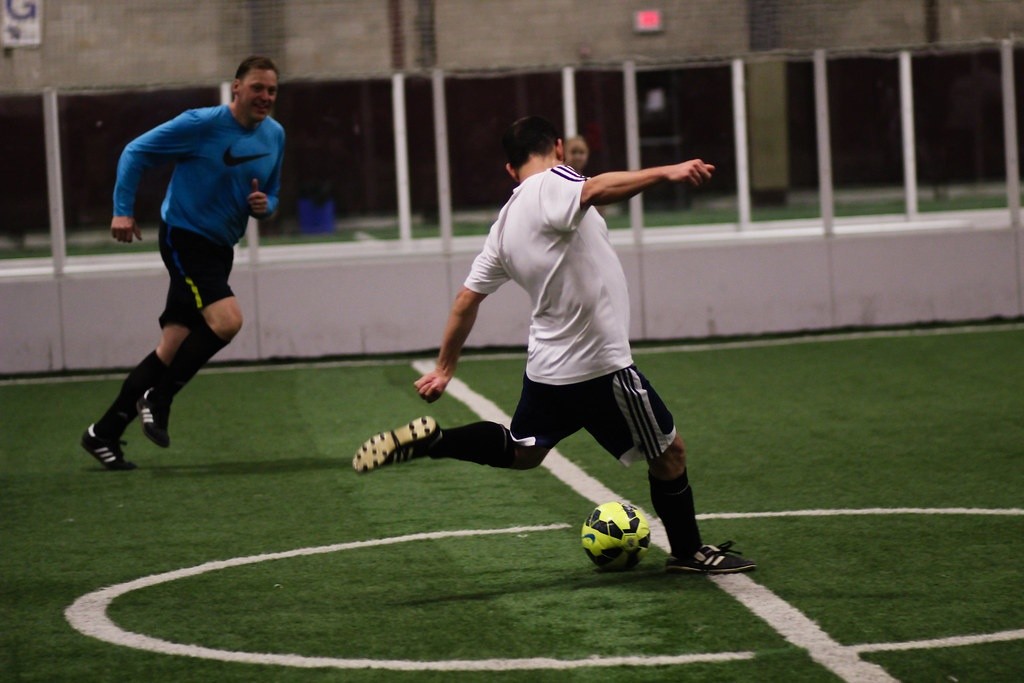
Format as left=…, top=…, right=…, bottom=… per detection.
left=561, top=132, right=588, bottom=172
left=78, top=55, right=287, bottom=470
left=350, top=116, right=759, bottom=575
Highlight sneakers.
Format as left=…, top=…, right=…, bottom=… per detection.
left=82, top=423, right=133, bottom=471
left=666, top=540, right=757, bottom=575
left=352, top=415, right=442, bottom=474
left=137, top=388, right=170, bottom=448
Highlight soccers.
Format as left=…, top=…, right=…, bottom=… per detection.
left=581, top=501, right=650, bottom=570
